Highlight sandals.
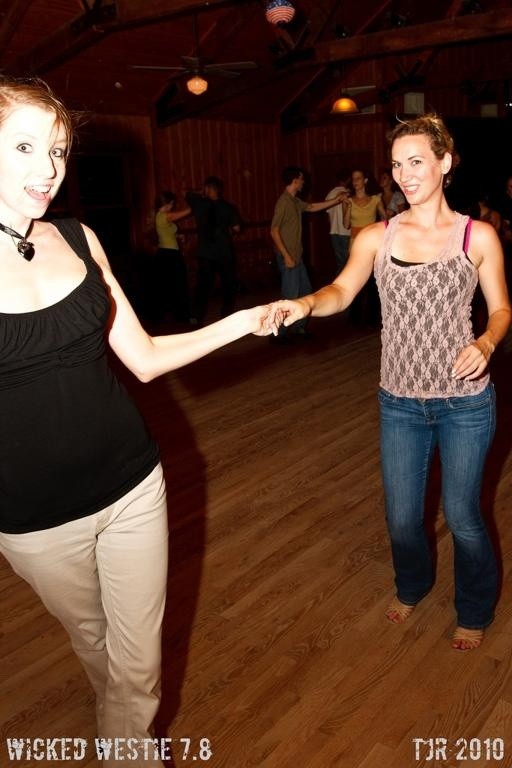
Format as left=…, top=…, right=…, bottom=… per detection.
left=385, top=594, right=414, bottom=624
left=451, top=626, right=484, bottom=653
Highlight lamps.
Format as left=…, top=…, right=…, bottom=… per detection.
left=330, top=84, right=359, bottom=115
left=188, top=61, right=206, bottom=95
left=266, top=0, right=295, bottom=26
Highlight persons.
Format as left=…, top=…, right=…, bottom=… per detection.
left=0, top=80, right=277, bottom=765
left=266, top=161, right=512, bottom=344
left=270, top=112, right=510, bottom=653
left=182, top=177, right=243, bottom=327
left=154, top=190, right=192, bottom=302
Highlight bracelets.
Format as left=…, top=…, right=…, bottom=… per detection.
left=477, top=337, right=495, bottom=353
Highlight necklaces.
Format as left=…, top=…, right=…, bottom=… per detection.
left=1, top=218, right=40, bottom=262
